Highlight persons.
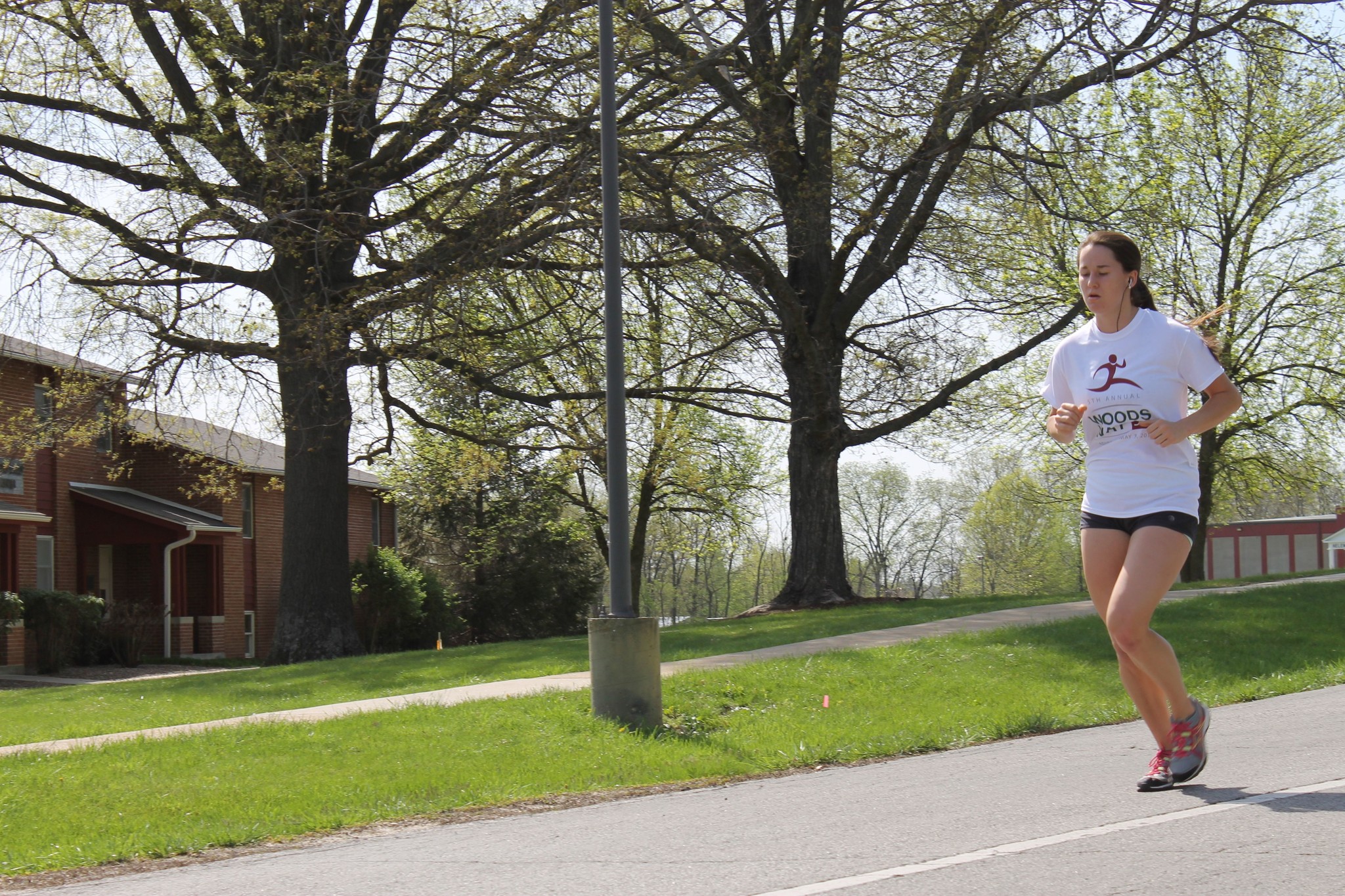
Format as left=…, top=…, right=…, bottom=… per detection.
left=1040, top=232, right=1242, bottom=792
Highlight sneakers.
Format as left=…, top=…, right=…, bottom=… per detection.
left=1166, top=702, right=1210, bottom=782
left=1137, top=741, right=1175, bottom=792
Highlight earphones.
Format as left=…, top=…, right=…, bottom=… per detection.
left=1128, top=278, right=1132, bottom=286
